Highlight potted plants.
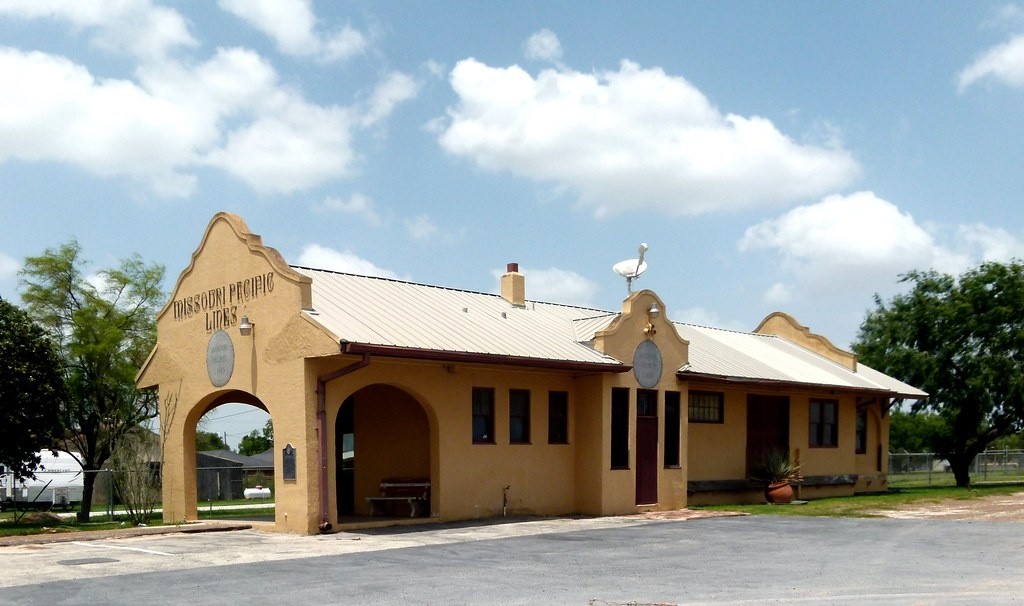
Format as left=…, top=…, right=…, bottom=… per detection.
left=757, top=444, right=803, bottom=503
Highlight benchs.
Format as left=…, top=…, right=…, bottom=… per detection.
left=364, top=477, right=431, bottom=517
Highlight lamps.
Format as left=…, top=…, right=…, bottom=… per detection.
left=240, top=315, right=256, bottom=335
left=646, top=300, right=660, bottom=318
